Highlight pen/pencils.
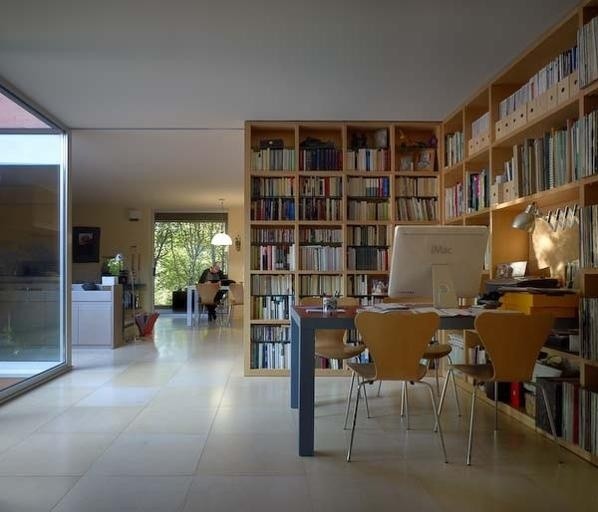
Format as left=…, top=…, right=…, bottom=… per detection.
left=323, top=292, right=326, bottom=296
left=334, top=291, right=340, bottom=297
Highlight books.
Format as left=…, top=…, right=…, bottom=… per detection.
left=495, top=19, right=598, bottom=195
left=445, top=112, right=490, bottom=219
left=316, top=357, right=343, bottom=370
left=448, top=298, right=598, bottom=457
left=299, top=149, right=344, bottom=306
left=420, top=337, right=437, bottom=369
left=582, top=204, right=598, bottom=268
left=362, top=302, right=409, bottom=314
left=346, top=148, right=390, bottom=305
left=347, top=329, right=369, bottom=364
left=250, top=147, right=296, bottom=369
left=395, top=178, right=437, bottom=220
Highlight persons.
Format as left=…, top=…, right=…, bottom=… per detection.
left=199, top=261, right=226, bottom=321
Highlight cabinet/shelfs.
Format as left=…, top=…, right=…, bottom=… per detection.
left=242, top=121, right=441, bottom=378
left=441, top=0, right=598, bottom=467
left=1, top=285, right=123, bottom=348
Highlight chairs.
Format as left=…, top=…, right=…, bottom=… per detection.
left=343, top=310, right=447, bottom=464
left=432, top=306, right=562, bottom=466
left=194, top=281, right=243, bottom=326
left=376, top=297, right=462, bottom=418
left=305, top=297, right=369, bottom=418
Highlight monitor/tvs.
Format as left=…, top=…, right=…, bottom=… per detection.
left=388, top=225, right=488, bottom=308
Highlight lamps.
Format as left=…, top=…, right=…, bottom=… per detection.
left=209, top=199, right=233, bottom=246
left=512, top=200, right=581, bottom=235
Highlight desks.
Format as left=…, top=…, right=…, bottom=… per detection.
left=186, top=285, right=229, bottom=326
left=287, top=305, right=578, bottom=456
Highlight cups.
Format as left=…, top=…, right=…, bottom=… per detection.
left=322, top=297, right=338, bottom=312
